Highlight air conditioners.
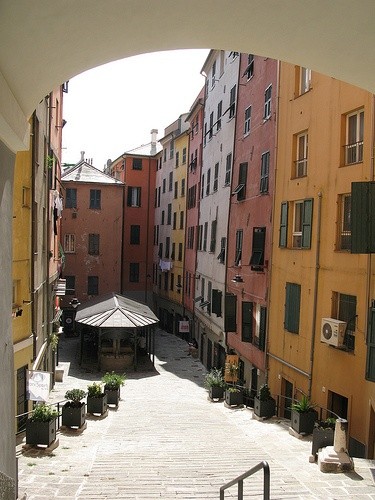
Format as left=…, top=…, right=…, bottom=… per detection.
left=320, top=318, right=347, bottom=347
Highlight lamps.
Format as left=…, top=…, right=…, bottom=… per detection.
left=60, top=297, right=81, bottom=308
left=231, top=259, right=268, bottom=287
left=176, top=274, right=200, bottom=290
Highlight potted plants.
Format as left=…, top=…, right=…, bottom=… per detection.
left=254, top=384, right=275, bottom=417
left=289, top=395, right=318, bottom=434
left=205, top=367, right=224, bottom=398
left=311, top=417, right=335, bottom=455
left=62, top=389, right=87, bottom=428
left=102, top=373, right=124, bottom=406
left=26, top=403, right=60, bottom=445
left=87, top=382, right=107, bottom=415
left=226, top=388, right=243, bottom=405
